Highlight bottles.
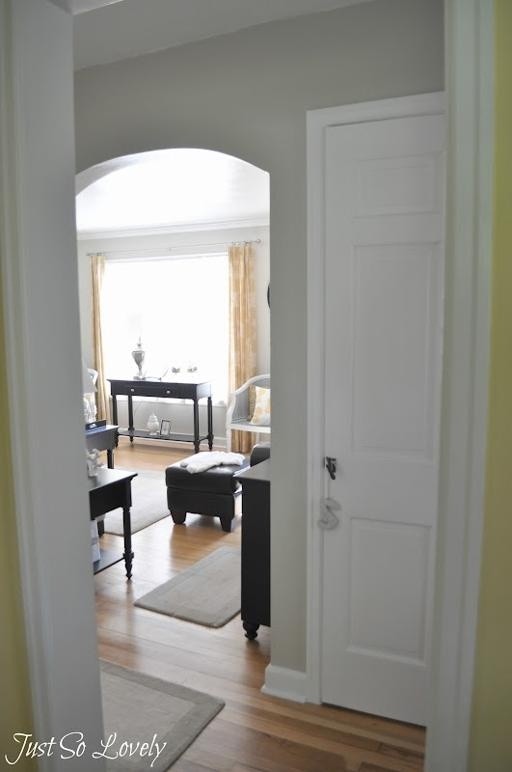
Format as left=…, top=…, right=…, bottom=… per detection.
left=147, top=413, right=159, bottom=436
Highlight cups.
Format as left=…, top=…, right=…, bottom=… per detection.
left=87, top=450, right=98, bottom=477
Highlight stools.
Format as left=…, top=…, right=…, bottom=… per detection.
left=166, top=452, right=251, bottom=532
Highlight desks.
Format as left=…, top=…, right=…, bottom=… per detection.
left=86, top=424, right=119, bottom=469
left=88, top=467, right=138, bottom=581
left=107, top=379, right=214, bottom=454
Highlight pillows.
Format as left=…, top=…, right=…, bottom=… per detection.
left=248, top=386, right=270, bottom=426
left=247, top=384, right=255, bottom=421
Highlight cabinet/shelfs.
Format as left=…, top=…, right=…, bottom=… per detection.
left=235, top=478, right=270, bottom=642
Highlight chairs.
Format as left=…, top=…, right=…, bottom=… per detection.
left=225, top=373, right=270, bottom=452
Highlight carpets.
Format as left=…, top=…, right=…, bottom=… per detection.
left=99, top=656, right=226, bottom=772
left=133, top=546, right=242, bottom=629
